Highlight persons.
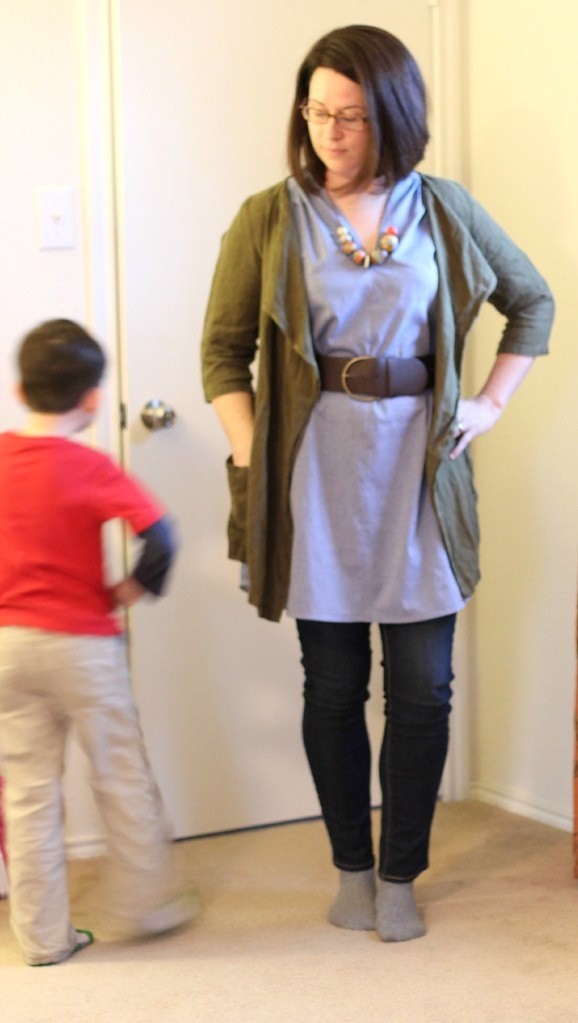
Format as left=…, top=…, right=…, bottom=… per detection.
left=0, top=320, right=198, bottom=965
left=203, top=23, right=555, bottom=942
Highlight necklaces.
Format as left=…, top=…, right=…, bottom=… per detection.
left=326, top=186, right=398, bottom=269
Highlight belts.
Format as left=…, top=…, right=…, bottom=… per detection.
left=318, top=355, right=434, bottom=402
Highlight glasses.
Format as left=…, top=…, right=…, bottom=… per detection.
left=298, top=97, right=369, bottom=133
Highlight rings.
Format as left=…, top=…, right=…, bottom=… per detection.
left=457, top=423, right=463, bottom=433
